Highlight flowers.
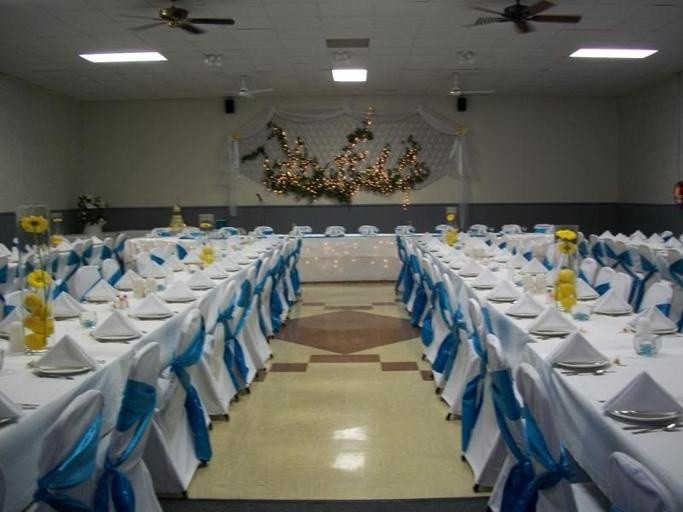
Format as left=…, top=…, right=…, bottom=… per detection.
left=19, top=214, right=53, bottom=354
left=197, top=223, right=212, bottom=264
left=549, top=229, right=576, bottom=313
left=445, top=213, right=457, bottom=246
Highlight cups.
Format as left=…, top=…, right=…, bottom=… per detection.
left=445, top=206, right=456, bottom=228
left=50, top=211, right=64, bottom=248
left=197, top=214, right=215, bottom=234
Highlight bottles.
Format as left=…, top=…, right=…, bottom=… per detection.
left=169, top=205, right=184, bottom=229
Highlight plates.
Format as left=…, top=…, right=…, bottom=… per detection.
left=292, top=225, right=378, bottom=236
left=391, top=222, right=683, bottom=435
left=0, top=224, right=283, bottom=422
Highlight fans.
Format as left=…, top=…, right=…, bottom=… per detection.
left=461, top=2, right=581, bottom=34
left=427, top=73, right=496, bottom=99
left=210, top=75, right=275, bottom=101
left=115, top=2, right=235, bottom=34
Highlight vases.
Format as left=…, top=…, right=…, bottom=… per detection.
left=444, top=206, right=458, bottom=247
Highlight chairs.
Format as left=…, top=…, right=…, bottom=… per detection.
left=290, top=226, right=380, bottom=234
